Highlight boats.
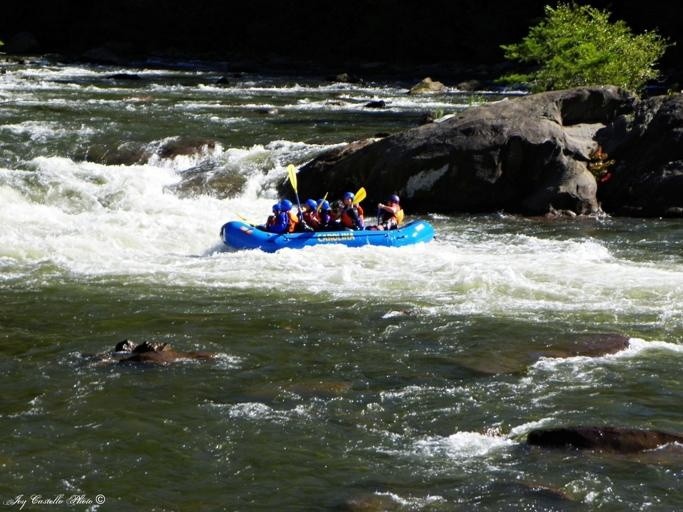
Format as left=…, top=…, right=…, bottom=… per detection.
left=219, top=220, right=435, bottom=253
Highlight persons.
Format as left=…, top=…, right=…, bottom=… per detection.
left=263, top=191, right=364, bottom=234
left=373, top=193, right=402, bottom=231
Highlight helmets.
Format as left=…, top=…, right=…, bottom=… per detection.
left=388, top=195, right=399, bottom=204
left=306, top=199, right=317, bottom=210
left=317, top=198, right=329, bottom=209
left=343, top=192, right=355, bottom=201
left=276, top=199, right=293, bottom=212
left=272, top=204, right=281, bottom=211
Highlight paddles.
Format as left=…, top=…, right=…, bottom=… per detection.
left=287, top=164, right=301, bottom=215
left=314, top=192, right=328, bottom=212
left=351, top=187, right=366, bottom=205
